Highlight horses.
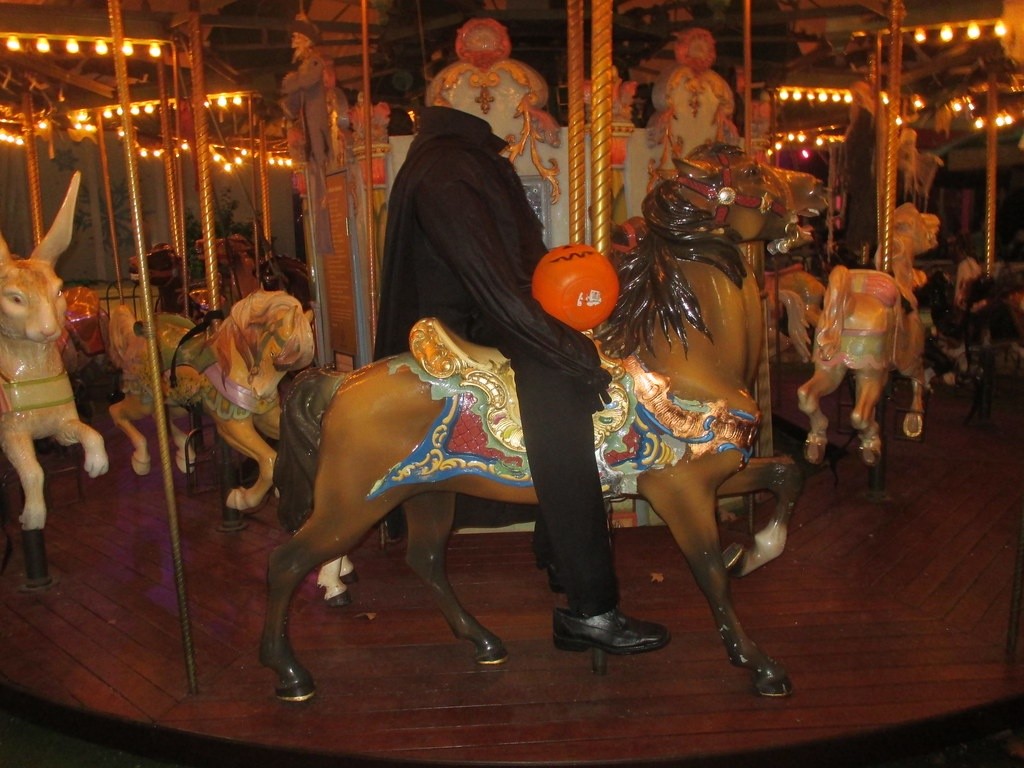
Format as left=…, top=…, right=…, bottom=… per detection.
left=109, top=289, right=319, bottom=511
left=797, top=203, right=940, bottom=464
left=260, top=126, right=831, bottom=701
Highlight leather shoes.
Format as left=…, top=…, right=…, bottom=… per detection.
left=547, top=565, right=563, bottom=593
left=551, top=606, right=671, bottom=655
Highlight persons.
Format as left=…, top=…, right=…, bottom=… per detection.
left=948, top=238, right=987, bottom=344
left=833, top=79, right=878, bottom=264
left=273, top=17, right=333, bottom=166
left=373, top=106, right=673, bottom=656
